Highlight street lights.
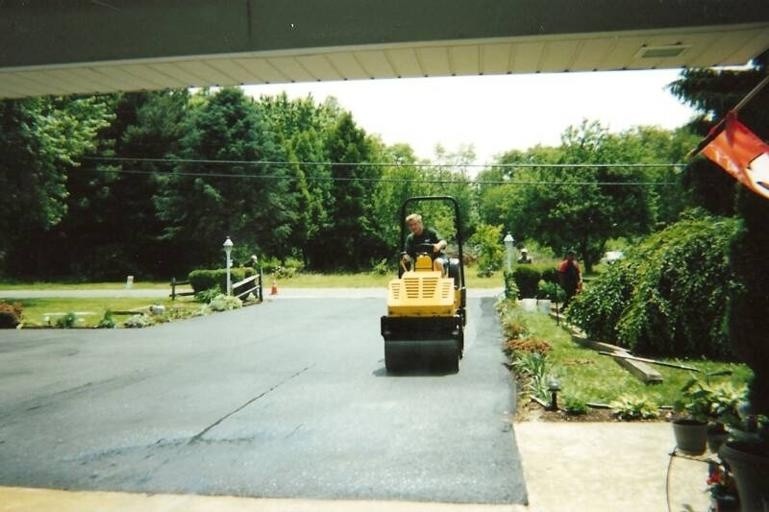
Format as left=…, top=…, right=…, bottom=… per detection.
left=505, top=235, right=514, bottom=271
left=224, top=237, right=233, bottom=293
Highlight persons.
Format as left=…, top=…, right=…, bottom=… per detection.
left=558, top=252, right=583, bottom=313
left=517, top=249, right=531, bottom=263
left=399, top=213, right=449, bottom=279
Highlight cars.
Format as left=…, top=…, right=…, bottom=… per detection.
left=600, top=252, right=623, bottom=264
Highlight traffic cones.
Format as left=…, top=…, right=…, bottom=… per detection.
left=271, top=278, right=278, bottom=295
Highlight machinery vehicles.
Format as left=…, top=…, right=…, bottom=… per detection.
left=380, top=195, right=467, bottom=371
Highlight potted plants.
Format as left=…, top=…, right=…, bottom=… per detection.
left=669, top=380, right=769, bottom=512
